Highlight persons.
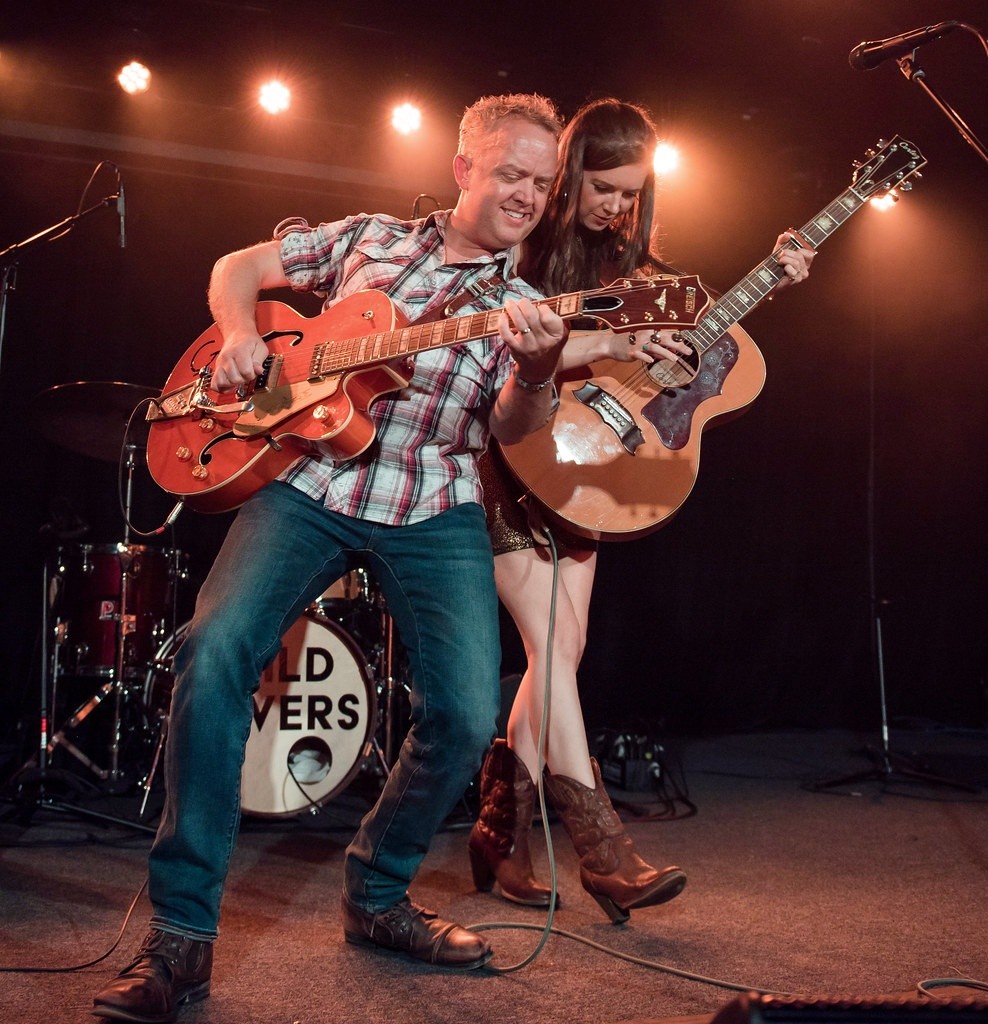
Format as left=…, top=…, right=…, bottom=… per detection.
left=89, top=93, right=563, bottom=1024
left=468, top=103, right=814, bottom=924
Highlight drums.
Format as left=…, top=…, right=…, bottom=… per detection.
left=144, top=605, right=381, bottom=821
left=43, top=538, right=191, bottom=681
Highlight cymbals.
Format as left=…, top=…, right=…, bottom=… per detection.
left=25, top=380, right=163, bottom=466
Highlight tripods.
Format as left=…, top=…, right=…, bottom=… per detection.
left=800, top=286, right=981, bottom=792
left=0, top=417, right=169, bottom=836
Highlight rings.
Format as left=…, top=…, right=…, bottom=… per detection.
left=642, top=342, right=649, bottom=350
left=520, top=327, right=530, bottom=335
left=790, top=270, right=801, bottom=280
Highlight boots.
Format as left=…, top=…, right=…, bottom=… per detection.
left=544, top=756, right=688, bottom=926
left=466, top=738, right=559, bottom=905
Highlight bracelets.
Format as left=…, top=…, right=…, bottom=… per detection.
left=513, top=362, right=556, bottom=391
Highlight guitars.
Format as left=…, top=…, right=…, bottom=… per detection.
left=491, top=133, right=929, bottom=543
left=144, top=272, right=711, bottom=514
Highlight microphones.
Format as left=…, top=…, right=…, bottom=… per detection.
left=849, top=20, right=958, bottom=73
left=75, top=160, right=119, bottom=226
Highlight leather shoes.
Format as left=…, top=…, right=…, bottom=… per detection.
left=89, top=927, right=215, bottom=1023
left=340, top=895, right=497, bottom=970
